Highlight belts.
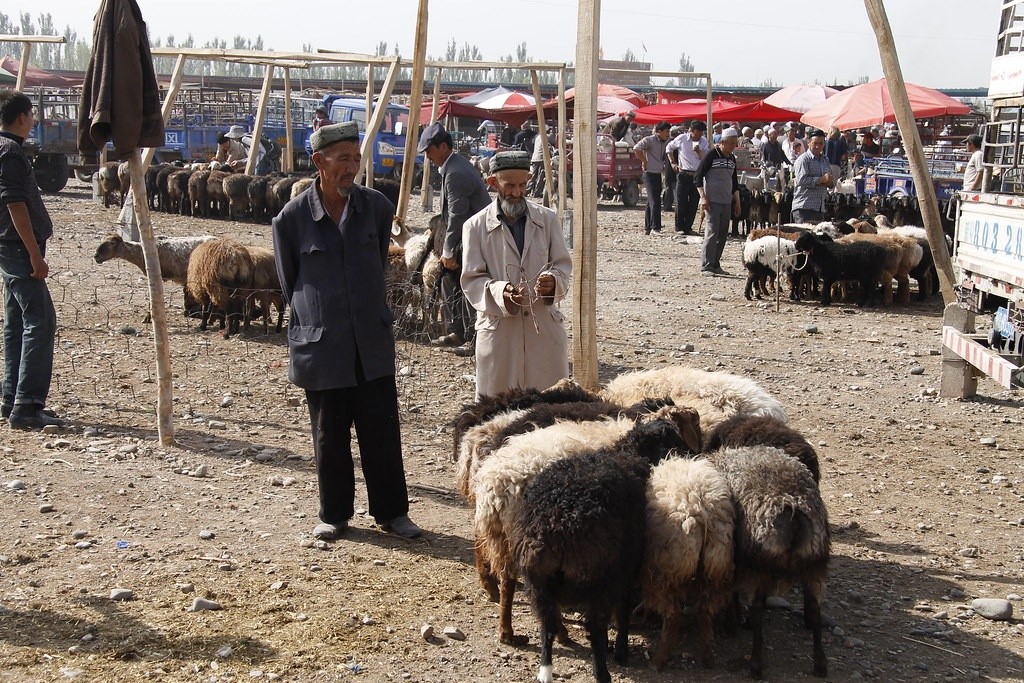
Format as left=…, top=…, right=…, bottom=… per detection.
left=680, top=169, right=695, bottom=176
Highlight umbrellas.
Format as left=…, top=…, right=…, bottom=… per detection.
left=376, top=74, right=970, bottom=155
left=0, top=56, right=84, bottom=141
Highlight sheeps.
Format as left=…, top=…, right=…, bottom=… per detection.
left=92, top=227, right=459, bottom=344
left=94, top=143, right=402, bottom=230
left=690, top=185, right=972, bottom=300
left=452, top=366, right=832, bottom=683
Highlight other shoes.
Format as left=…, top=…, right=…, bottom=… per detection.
left=453, top=341, right=475, bottom=355
left=432, top=333, right=464, bottom=346
left=313, top=518, right=350, bottom=538
left=649, top=229, right=663, bottom=238
left=1, top=403, right=56, bottom=417
left=714, top=270, right=730, bottom=275
left=701, top=271, right=714, bottom=277
left=9, top=413, right=61, bottom=428
left=676, top=230, right=698, bottom=235
left=383, top=512, right=424, bottom=541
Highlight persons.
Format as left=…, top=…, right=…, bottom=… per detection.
left=415, top=121, right=493, bottom=356
left=212, top=110, right=632, bottom=202
left=0, top=88, right=65, bottom=428
left=273, top=121, right=436, bottom=541
left=960, top=135, right=985, bottom=193
left=460, top=152, right=573, bottom=403
left=635, top=123, right=950, bottom=277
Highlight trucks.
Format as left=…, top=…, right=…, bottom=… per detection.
left=249, top=94, right=425, bottom=193
left=943, top=0, right=1024, bottom=396
left=851, top=143, right=1006, bottom=209
left=0, top=82, right=253, bottom=193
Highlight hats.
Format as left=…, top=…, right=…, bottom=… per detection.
left=224, top=125, right=244, bottom=139
left=721, top=128, right=737, bottom=137
left=742, top=121, right=797, bottom=135
left=309, top=121, right=359, bottom=151
left=316, top=105, right=328, bottom=115
left=627, top=110, right=636, bottom=118
left=962, top=134, right=981, bottom=147
left=545, top=124, right=550, bottom=131
left=216, top=131, right=229, bottom=145
left=488, top=150, right=531, bottom=174
left=691, top=120, right=707, bottom=131
left=417, top=120, right=445, bottom=153
left=809, top=129, right=825, bottom=138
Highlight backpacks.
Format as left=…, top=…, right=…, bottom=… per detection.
left=241, top=133, right=281, bottom=161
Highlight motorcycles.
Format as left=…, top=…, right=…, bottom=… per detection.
left=541, top=132, right=645, bottom=208
